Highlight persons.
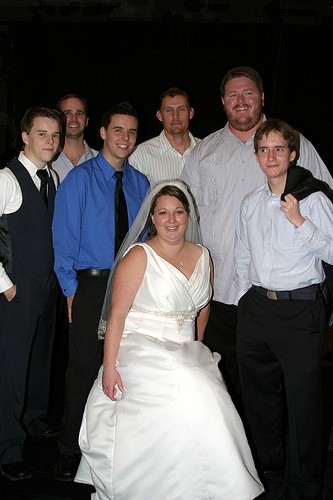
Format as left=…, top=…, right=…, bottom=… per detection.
left=233, top=119, right=333, bottom=500
left=47, top=94, right=100, bottom=184
left=181, top=66, right=333, bottom=483
left=0, top=107, right=60, bottom=481
left=126, top=88, right=203, bottom=187
left=52, top=102, right=151, bottom=478
left=73, top=185, right=264, bottom=500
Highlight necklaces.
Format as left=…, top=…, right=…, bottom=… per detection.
left=152, top=236, right=186, bottom=267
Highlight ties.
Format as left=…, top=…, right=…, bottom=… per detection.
left=36, top=169, right=49, bottom=208
left=114, top=171, right=129, bottom=259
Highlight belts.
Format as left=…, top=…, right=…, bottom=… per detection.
left=253, top=284, right=322, bottom=300
left=76, top=269, right=110, bottom=278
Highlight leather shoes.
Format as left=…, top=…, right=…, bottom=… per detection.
left=52, top=455, right=81, bottom=482
left=0, top=462, right=31, bottom=481
left=34, top=421, right=61, bottom=437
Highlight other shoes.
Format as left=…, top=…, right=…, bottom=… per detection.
left=255, top=463, right=284, bottom=475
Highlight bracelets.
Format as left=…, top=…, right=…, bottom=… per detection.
left=102, top=366, right=116, bottom=371
